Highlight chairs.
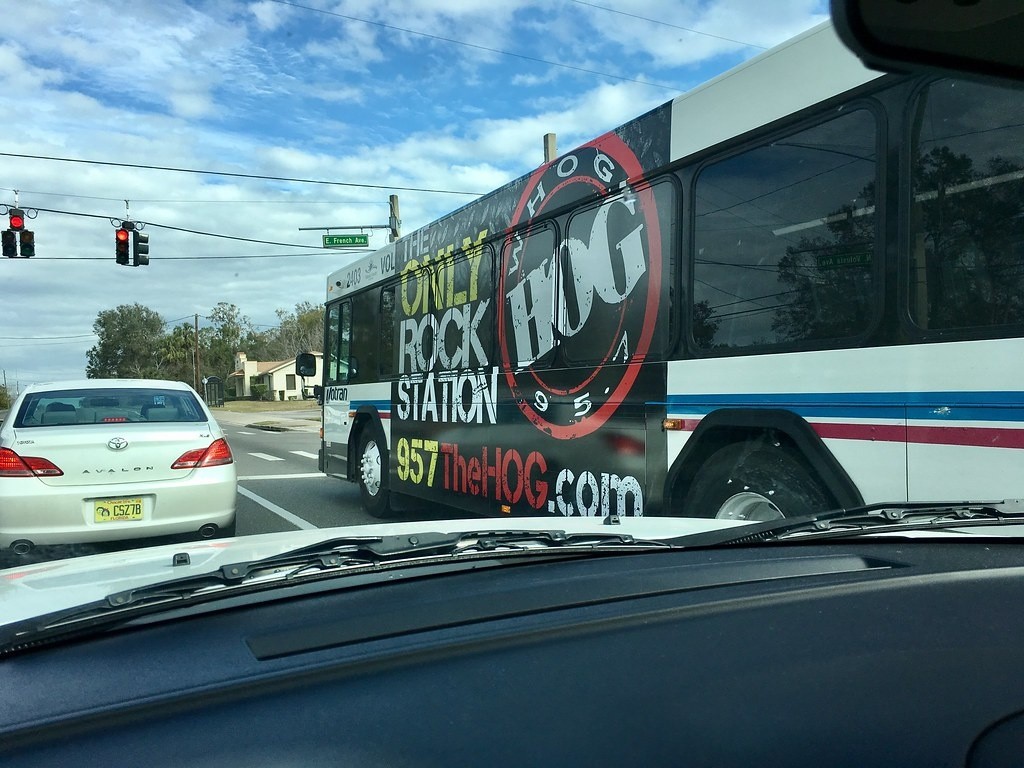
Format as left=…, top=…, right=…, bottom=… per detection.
left=141, top=404, right=180, bottom=421
left=941, top=233, right=1024, bottom=324
left=41, top=402, right=77, bottom=425
left=744, top=237, right=878, bottom=336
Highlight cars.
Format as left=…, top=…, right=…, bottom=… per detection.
left=0, top=378, right=237, bottom=554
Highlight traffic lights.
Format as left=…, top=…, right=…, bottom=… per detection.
left=19, top=229, right=35, bottom=258
left=1, top=231, right=17, bottom=258
left=115, top=228, right=129, bottom=265
left=133, top=230, right=149, bottom=267
left=9, top=209, right=24, bottom=231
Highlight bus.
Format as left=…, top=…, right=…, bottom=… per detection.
left=295, top=18, right=1024, bottom=517
